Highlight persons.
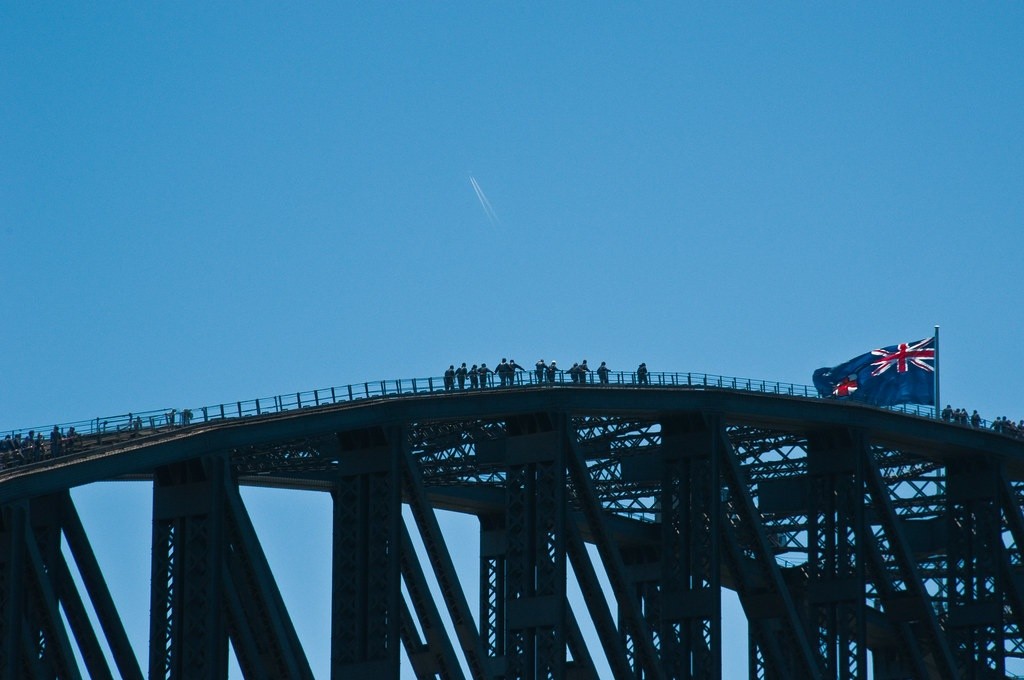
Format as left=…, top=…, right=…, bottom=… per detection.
left=637, top=363, right=648, bottom=384
left=535, top=359, right=548, bottom=382
left=493, top=358, right=526, bottom=386
left=597, top=361, right=612, bottom=383
left=941, top=403, right=981, bottom=428
left=990, top=415, right=1024, bottom=440
left=0, top=426, right=77, bottom=452
left=562, top=357, right=591, bottom=393
left=546, top=361, right=562, bottom=382
left=445, top=363, right=493, bottom=390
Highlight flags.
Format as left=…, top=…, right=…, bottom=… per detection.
left=811, top=336, right=935, bottom=407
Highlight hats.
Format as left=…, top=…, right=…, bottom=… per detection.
left=552, top=361, right=556, bottom=364
